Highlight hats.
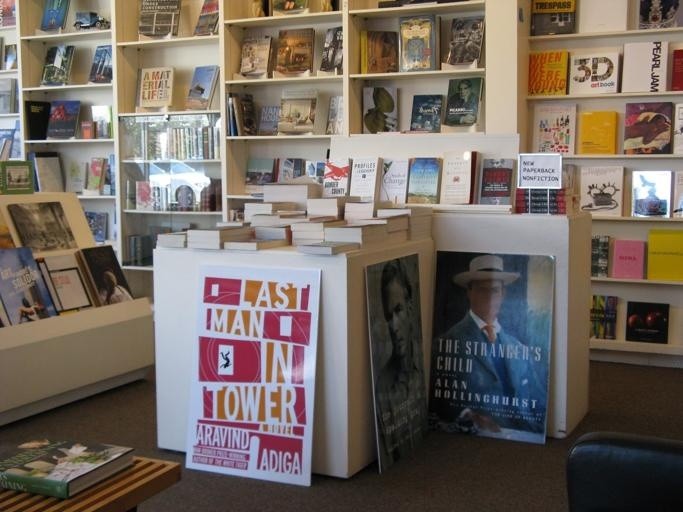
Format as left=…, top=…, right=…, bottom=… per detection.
left=454, top=256, right=521, bottom=287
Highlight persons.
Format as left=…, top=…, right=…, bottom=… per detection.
left=377, top=259, right=423, bottom=458
left=434, top=251, right=545, bottom=439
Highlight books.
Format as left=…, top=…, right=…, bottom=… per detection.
left=226, top=0, right=343, bottom=218
left=1, top=0, right=133, bottom=327
left=0, top=438, right=134, bottom=499
left=126, top=1, right=219, bottom=264
left=160, top=183, right=433, bottom=255
left=530, top=3, right=680, bottom=346
left=268, top=153, right=582, bottom=216
left=360, top=0, right=485, bottom=134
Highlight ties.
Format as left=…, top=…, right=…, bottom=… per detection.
left=484, top=324, right=497, bottom=341
